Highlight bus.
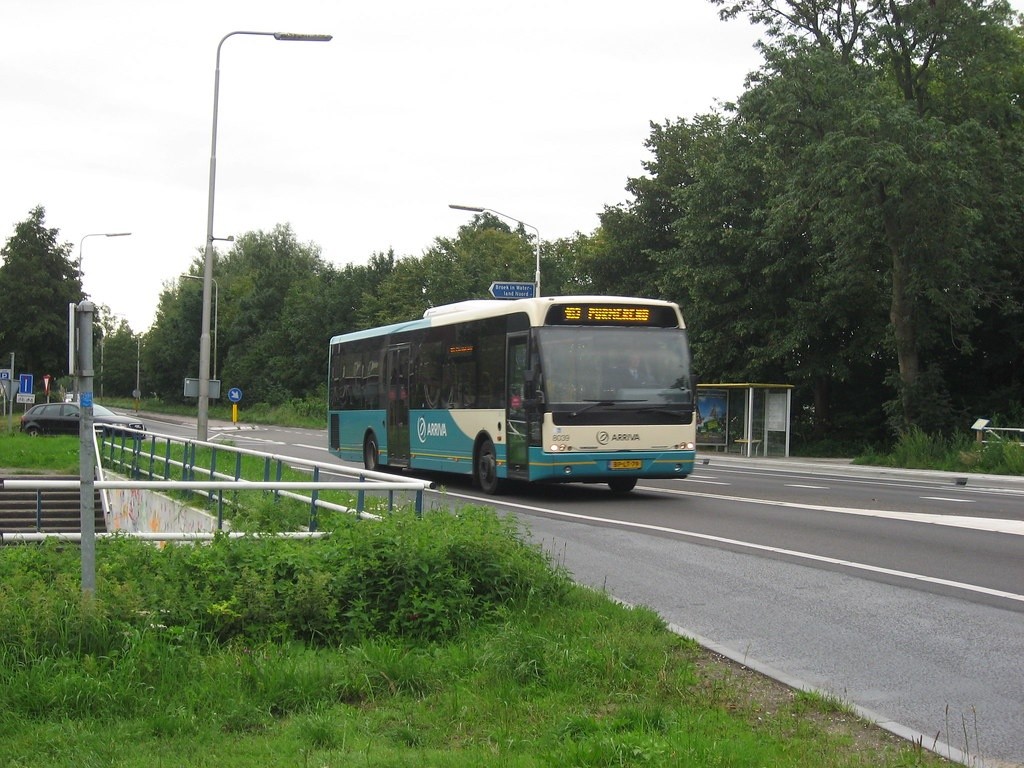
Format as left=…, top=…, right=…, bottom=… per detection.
left=326, top=280, right=699, bottom=498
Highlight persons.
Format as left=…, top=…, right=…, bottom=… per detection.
left=620, top=353, right=644, bottom=388
left=467, top=371, right=494, bottom=408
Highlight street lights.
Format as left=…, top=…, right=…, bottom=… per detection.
left=182, top=273, right=219, bottom=410
left=78, top=232, right=131, bottom=291
left=194, top=30, right=335, bottom=442
left=449, top=203, right=542, bottom=297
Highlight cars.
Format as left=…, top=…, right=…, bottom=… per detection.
left=19, top=401, right=148, bottom=439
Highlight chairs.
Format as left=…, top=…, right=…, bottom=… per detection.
left=335, top=361, right=510, bottom=415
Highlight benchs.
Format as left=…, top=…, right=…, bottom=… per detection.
left=734, top=438, right=762, bottom=456
